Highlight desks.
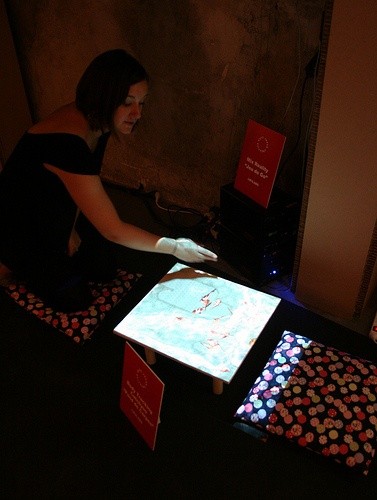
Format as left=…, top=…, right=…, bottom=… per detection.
left=114, top=262, right=282, bottom=395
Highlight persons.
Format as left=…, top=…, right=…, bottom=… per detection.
left=0, top=47, right=222, bottom=276
